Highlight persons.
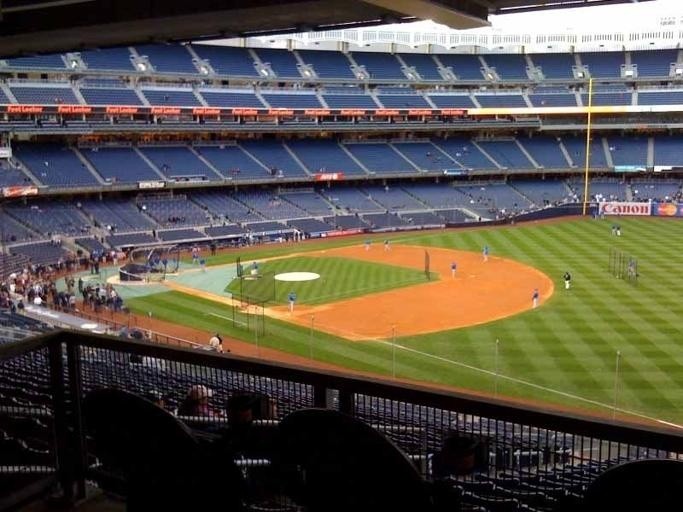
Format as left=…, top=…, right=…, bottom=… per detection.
left=1, top=144, right=681, bottom=362
left=143, top=383, right=278, bottom=435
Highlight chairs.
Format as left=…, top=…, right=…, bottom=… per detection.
left=72, top=392, right=243, bottom=510
left=259, top=411, right=452, bottom=510
left=574, top=458, right=682, bottom=512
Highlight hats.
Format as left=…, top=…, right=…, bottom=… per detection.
left=144, top=389, right=169, bottom=401
left=187, top=384, right=216, bottom=400
